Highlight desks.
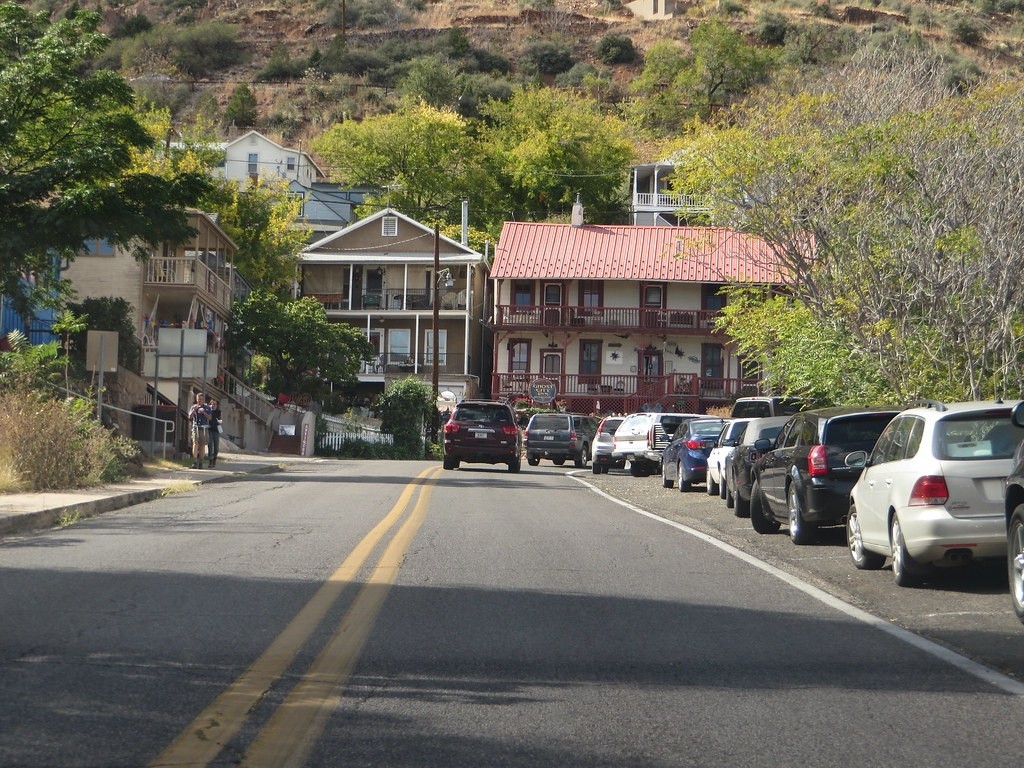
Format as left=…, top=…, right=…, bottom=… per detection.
left=514, top=379, right=530, bottom=394
left=398, top=363, right=423, bottom=373
left=599, top=385, right=612, bottom=394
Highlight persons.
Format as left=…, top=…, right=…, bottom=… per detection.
left=208, top=400, right=222, bottom=468
left=189, top=393, right=212, bottom=469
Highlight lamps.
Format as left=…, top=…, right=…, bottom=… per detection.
left=377, top=266, right=386, bottom=277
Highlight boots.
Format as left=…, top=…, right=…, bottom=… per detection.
left=213, top=458, right=216, bottom=467
left=208, top=459, right=212, bottom=467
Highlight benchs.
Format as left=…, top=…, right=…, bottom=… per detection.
left=670, top=312, right=693, bottom=328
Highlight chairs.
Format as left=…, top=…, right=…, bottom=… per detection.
left=614, top=381, right=625, bottom=395
left=151, top=261, right=175, bottom=281
left=587, top=378, right=597, bottom=394
left=502, top=377, right=513, bottom=392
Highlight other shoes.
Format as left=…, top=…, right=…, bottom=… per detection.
left=189, top=463, right=197, bottom=468
left=198, top=463, right=202, bottom=468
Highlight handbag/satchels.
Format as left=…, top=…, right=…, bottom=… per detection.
left=217, top=425, right=224, bottom=433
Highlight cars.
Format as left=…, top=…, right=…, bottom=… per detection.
left=658, top=417, right=730, bottom=491
left=706, top=417, right=761, bottom=499
left=749, top=406, right=915, bottom=543
left=724, top=417, right=794, bottom=520
left=1004, top=401, right=1024, bottom=622
left=591, top=412, right=635, bottom=473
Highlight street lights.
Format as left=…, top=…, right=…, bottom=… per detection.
left=432, top=268, right=457, bottom=442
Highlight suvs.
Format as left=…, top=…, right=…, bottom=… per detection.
left=522, top=411, right=601, bottom=468
left=612, top=413, right=720, bottom=477
left=730, top=397, right=835, bottom=419
left=441, top=398, right=524, bottom=472
left=844, top=399, right=1024, bottom=587
